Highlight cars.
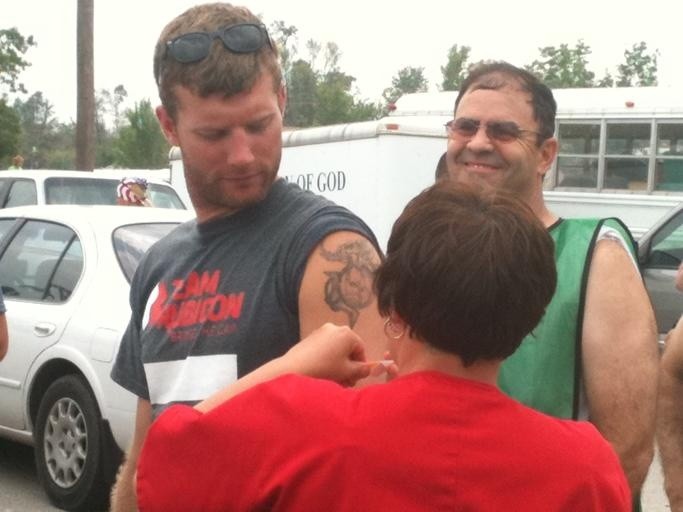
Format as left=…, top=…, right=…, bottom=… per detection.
left=634, top=206, right=683, bottom=354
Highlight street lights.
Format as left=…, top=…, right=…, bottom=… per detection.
left=30, top=146, right=39, bottom=169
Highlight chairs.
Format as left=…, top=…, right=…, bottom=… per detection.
left=1, top=260, right=82, bottom=301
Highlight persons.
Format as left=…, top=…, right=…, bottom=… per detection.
left=655, top=258, right=681, bottom=510
left=439, top=58, right=659, bottom=512
left=132, top=153, right=635, bottom=510
left=105, top=1, right=390, bottom=510
left=116, top=176, right=152, bottom=208
left=6, top=154, right=24, bottom=170
left=0, top=285, right=9, bottom=362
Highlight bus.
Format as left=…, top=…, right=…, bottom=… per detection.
left=386, top=87, right=682, bottom=245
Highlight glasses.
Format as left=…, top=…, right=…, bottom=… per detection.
left=444, top=117, right=547, bottom=144
left=154, top=22, right=279, bottom=65
